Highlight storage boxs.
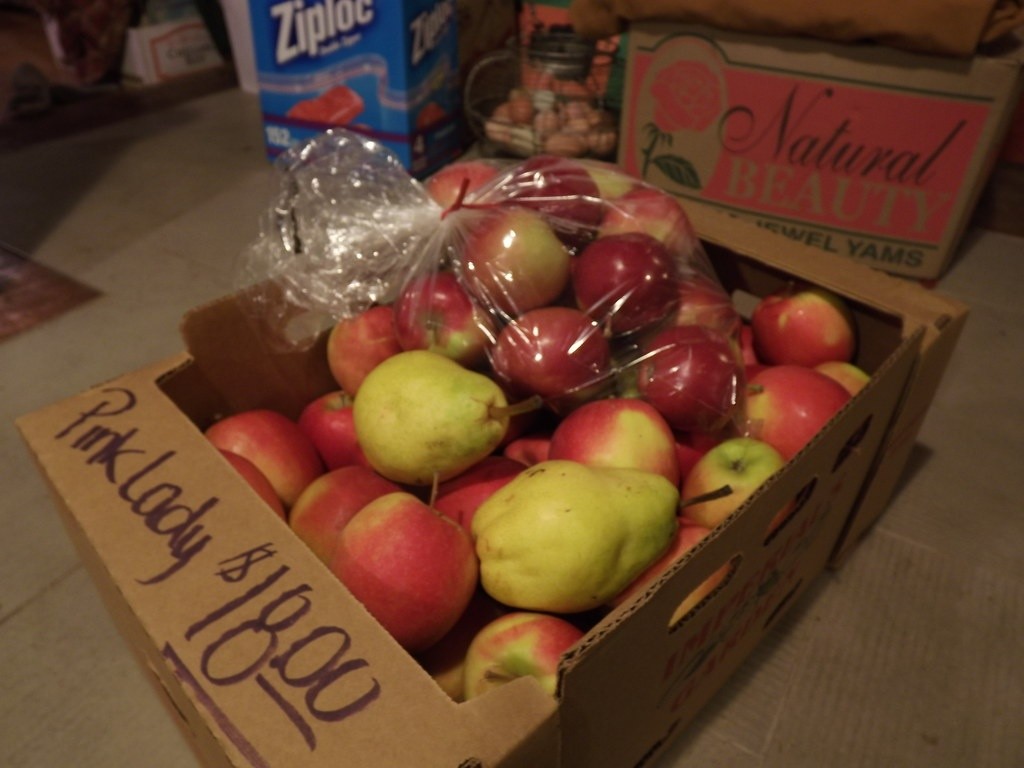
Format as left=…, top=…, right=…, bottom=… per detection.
left=614, top=24, right=1024, bottom=291
left=17, top=158, right=970, bottom=768
left=572, top=0, right=1024, bottom=56
left=248, top=0, right=516, bottom=189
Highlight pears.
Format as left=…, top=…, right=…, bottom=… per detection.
left=469, top=457, right=733, bottom=614
left=353, top=350, right=542, bottom=486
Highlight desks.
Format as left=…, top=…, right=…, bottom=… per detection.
left=0, top=0, right=1024, bottom=768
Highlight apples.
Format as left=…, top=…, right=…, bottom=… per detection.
left=194, top=153, right=874, bottom=708
